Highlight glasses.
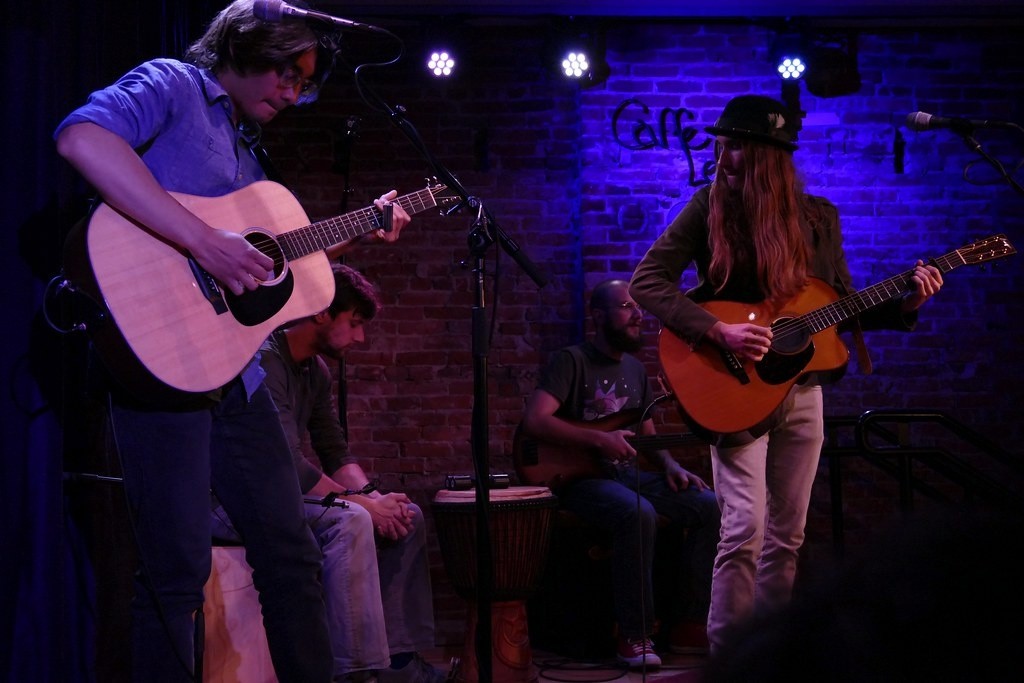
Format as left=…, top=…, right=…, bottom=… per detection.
left=277, top=67, right=318, bottom=97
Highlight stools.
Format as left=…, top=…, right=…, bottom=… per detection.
left=192, top=537, right=245, bottom=683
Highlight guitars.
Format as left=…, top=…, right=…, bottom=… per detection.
left=656, top=234, right=1020, bottom=449
left=513, top=409, right=707, bottom=494
left=82, top=173, right=466, bottom=413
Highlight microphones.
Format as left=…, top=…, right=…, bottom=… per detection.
left=905, top=112, right=1007, bottom=133
left=253, top=0, right=369, bottom=33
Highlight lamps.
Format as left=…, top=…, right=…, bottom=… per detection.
left=428, top=52, right=454, bottom=77
left=560, top=50, right=596, bottom=88
left=773, top=40, right=808, bottom=82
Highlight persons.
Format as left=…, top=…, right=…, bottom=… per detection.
left=525, top=279, right=719, bottom=667
left=631, top=95, right=943, bottom=663
left=53, top=1, right=339, bottom=683
left=260, top=263, right=462, bottom=683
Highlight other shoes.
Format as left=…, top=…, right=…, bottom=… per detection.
left=379, top=654, right=451, bottom=682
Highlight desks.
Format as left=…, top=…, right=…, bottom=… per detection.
left=433, top=487, right=555, bottom=682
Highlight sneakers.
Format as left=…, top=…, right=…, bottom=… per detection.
left=619, top=634, right=664, bottom=666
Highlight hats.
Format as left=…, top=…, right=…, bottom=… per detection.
left=706, top=94, right=802, bottom=153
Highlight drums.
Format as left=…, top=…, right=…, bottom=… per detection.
left=431, top=486, right=558, bottom=683
